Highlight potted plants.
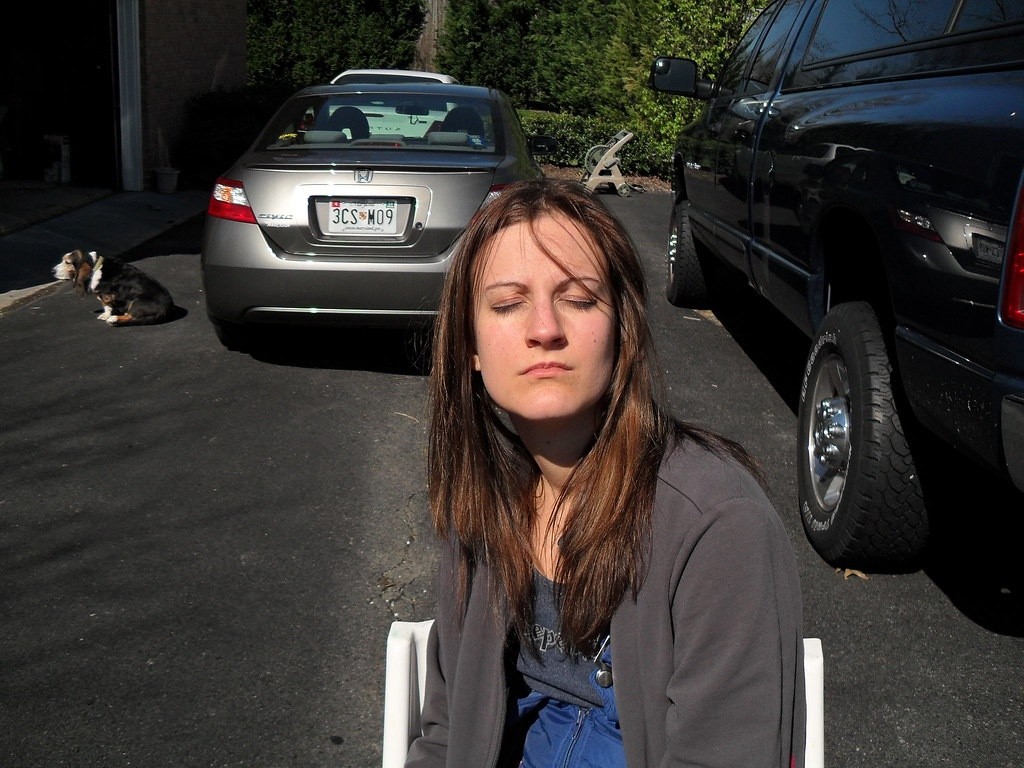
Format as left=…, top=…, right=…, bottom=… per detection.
left=243, top=71, right=284, bottom=125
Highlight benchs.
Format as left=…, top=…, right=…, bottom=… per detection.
left=302, top=130, right=469, bottom=145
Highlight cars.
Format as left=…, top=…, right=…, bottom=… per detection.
left=201, top=81, right=557, bottom=351
left=296, top=69, right=463, bottom=140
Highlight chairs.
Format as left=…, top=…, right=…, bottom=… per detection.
left=382, top=616, right=824, bottom=768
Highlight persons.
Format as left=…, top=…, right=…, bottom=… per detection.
left=405, top=178, right=807, bottom=768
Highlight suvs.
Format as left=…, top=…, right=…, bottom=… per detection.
left=646, top=0, right=1023, bottom=574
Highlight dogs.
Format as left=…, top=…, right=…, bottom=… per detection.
left=50, top=248, right=175, bottom=328
